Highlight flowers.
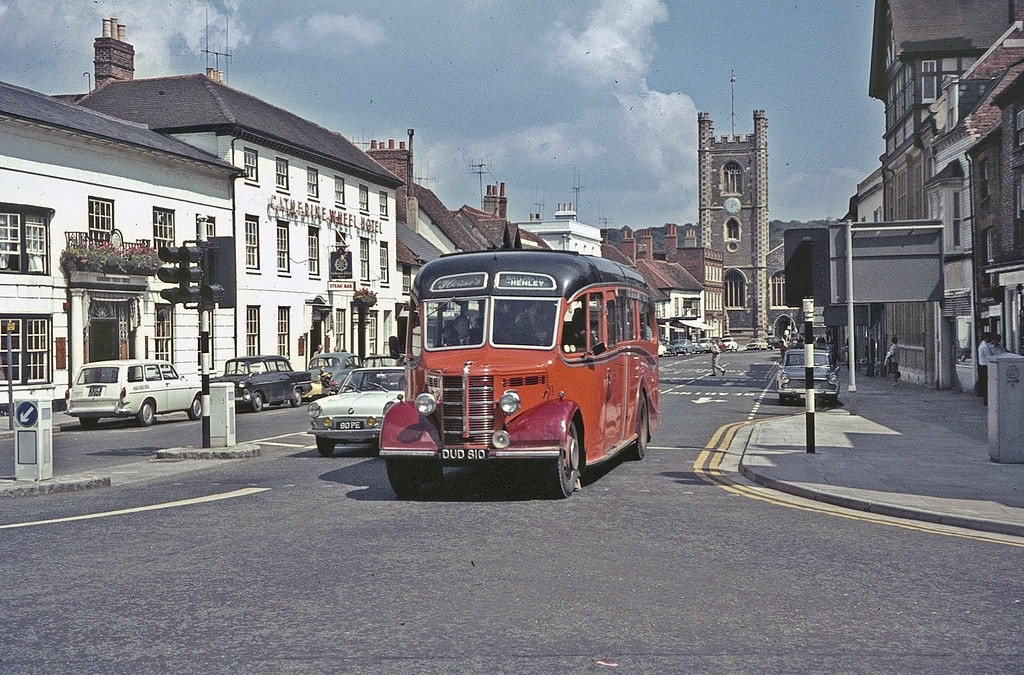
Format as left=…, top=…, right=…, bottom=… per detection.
left=353, top=288, right=378, bottom=306
left=60, top=240, right=164, bottom=269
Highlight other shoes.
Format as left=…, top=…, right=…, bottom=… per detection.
left=722, top=369, right=726, bottom=376
left=709, top=374, right=716, bottom=376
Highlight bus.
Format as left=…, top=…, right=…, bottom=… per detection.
left=378, top=247, right=659, bottom=499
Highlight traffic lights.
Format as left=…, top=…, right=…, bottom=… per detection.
left=157, top=236, right=238, bottom=308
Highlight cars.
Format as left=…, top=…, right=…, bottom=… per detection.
left=209, top=354, right=313, bottom=413
left=299, top=352, right=405, bottom=402
left=746, top=338, right=769, bottom=351
left=657, top=340, right=667, bottom=357
left=692, top=337, right=739, bottom=354
left=763, top=336, right=784, bottom=350
left=774, top=347, right=840, bottom=406
left=666, top=338, right=695, bottom=356
left=307, top=366, right=406, bottom=457
left=66, top=359, right=202, bottom=430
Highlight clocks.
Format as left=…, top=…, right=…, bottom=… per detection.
left=724, top=198, right=741, bottom=215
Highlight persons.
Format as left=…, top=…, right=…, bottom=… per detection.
left=313, top=344, right=325, bottom=356
left=978, top=332, right=1002, bottom=406
left=710, top=340, right=726, bottom=376
left=334, top=346, right=341, bottom=353
left=441, top=320, right=455, bottom=347
left=884, top=338, right=901, bottom=387
left=452, top=316, right=477, bottom=347
left=780, top=341, right=788, bottom=360
